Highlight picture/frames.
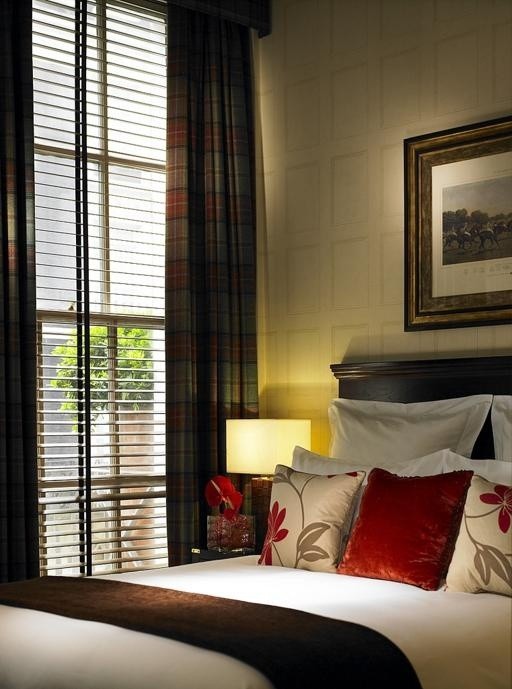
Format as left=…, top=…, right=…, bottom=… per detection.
left=402, top=116, right=511, bottom=331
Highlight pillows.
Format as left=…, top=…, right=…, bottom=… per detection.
left=490, top=395, right=511, bottom=461
left=258, top=464, right=366, bottom=574
left=337, top=468, right=473, bottom=592
left=288, top=439, right=450, bottom=531
left=443, top=475, right=512, bottom=597
left=442, top=448, right=512, bottom=492
left=328, top=394, right=493, bottom=459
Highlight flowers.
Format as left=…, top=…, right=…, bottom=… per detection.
left=205, top=477, right=243, bottom=520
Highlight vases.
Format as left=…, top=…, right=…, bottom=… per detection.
left=207, top=517, right=251, bottom=550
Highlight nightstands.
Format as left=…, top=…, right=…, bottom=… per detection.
left=190, top=545, right=261, bottom=563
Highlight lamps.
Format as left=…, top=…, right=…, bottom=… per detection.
left=226, top=418, right=311, bottom=547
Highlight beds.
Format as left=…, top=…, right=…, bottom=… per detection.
left=0, top=357, right=510, bottom=688
